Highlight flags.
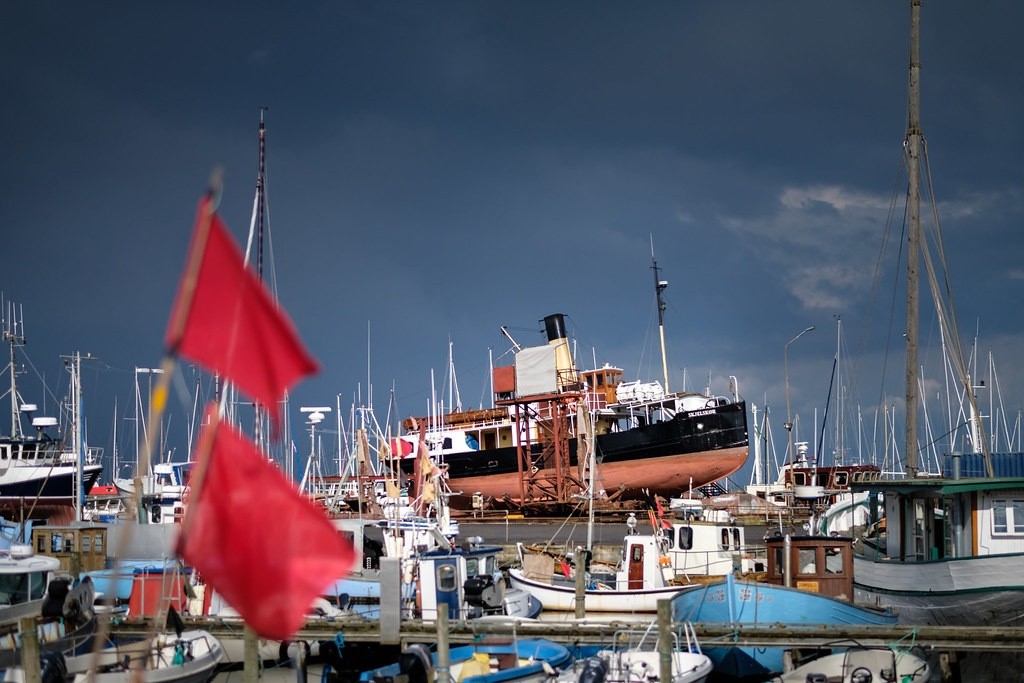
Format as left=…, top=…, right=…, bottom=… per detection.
left=170, top=402, right=360, bottom=640
left=419, top=454, right=434, bottom=475
left=390, top=438, right=411, bottom=457
left=422, top=481, right=434, bottom=502
left=386, top=479, right=399, bottom=497
left=660, top=519, right=673, bottom=529
left=656, top=498, right=665, bottom=518
left=648, top=510, right=657, bottom=525
left=167, top=199, right=324, bottom=441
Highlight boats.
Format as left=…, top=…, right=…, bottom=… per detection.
left=0, top=0, right=1023, bottom=683
